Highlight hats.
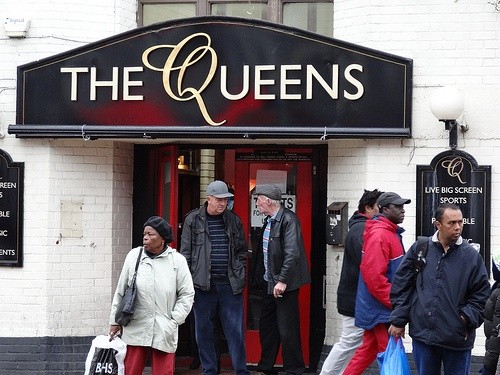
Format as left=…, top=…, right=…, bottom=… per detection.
left=377, top=192, right=411, bottom=208
left=206, top=180, right=234, bottom=198
left=144, top=216, right=174, bottom=243
left=253, top=185, right=281, bottom=200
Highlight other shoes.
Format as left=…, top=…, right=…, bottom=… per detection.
left=245, top=365, right=275, bottom=375
left=190, top=356, right=201, bottom=369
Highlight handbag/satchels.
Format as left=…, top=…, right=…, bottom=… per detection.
left=84, top=335, right=128, bottom=375
left=377, top=335, right=412, bottom=375
left=115, top=283, right=136, bottom=327
